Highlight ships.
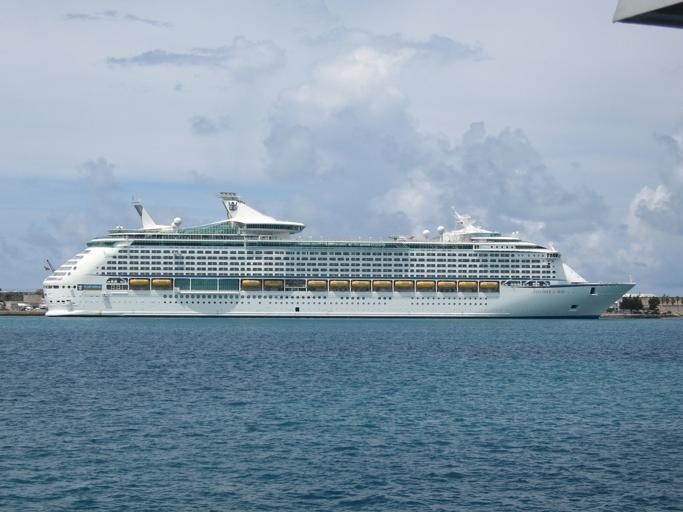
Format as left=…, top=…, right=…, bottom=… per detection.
left=40, top=192, right=636, bottom=320
